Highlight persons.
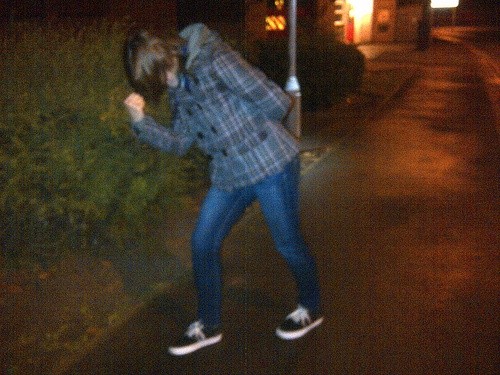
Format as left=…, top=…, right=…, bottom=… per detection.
left=123, top=21, right=325, bottom=356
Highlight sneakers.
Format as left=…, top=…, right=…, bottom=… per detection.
left=168, top=321, right=224, bottom=355
left=274, top=305, right=324, bottom=340
